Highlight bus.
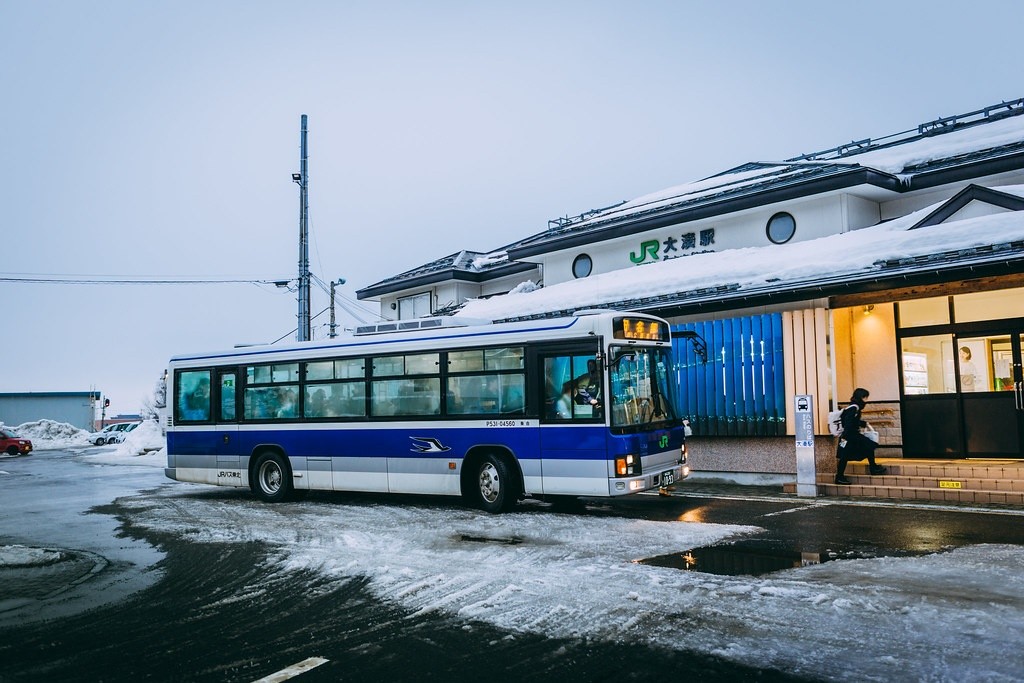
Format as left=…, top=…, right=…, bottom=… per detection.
left=164, top=309, right=708, bottom=514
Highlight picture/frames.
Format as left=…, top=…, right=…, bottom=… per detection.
left=941, top=338, right=990, bottom=393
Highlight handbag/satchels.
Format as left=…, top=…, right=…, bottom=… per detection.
left=861, top=423, right=878, bottom=444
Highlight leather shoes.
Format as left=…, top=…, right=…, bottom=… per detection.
left=871, top=466, right=886, bottom=475
left=835, top=474, right=851, bottom=485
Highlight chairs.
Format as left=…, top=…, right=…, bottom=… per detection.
left=182, top=385, right=523, bottom=420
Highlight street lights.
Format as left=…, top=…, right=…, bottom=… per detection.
left=329, top=277, right=346, bottom=339
left=292, top=173, right=311, bottom=342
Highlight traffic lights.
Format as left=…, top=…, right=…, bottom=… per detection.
left=105, top=399, right=109, bottom=406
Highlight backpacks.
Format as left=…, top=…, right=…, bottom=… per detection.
left=828, top=404, right=859, bottom=437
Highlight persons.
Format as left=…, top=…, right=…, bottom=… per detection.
left=304, top=383, right=365, bottom=415
left=188, top=377, right=210, bottom=409
left=835, top=388, right=886, bottom=485
left=258, top=387, right=298, bottom=418
left=959, top=347, right=975, bottom=375
left=556, top=381, right=577, bottom=419
left=481, top=375, right=498, bottom=409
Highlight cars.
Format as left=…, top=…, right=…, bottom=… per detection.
left=104, top=423, right=130, bottom=444
left=114, top=424, right=139, bottom=443
left=0, top=429, right=33, bottom=456
left=89, top=424, right=115, bottom=446
